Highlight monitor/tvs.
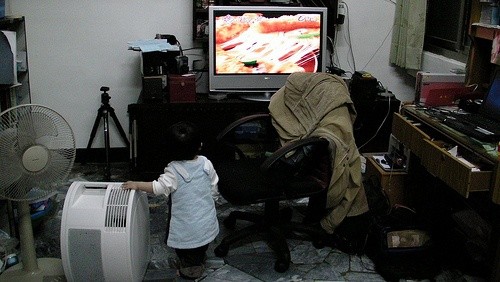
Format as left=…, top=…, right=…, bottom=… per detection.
left=209, top=6, right=327, bottom=101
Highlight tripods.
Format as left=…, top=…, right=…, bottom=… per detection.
left=80, top=86, right=131, bottom=182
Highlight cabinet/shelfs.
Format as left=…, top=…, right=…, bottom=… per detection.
left=128, top=94, right=401, bottom=196
left=365, top=156, right=408, bottom=206
left=192, top=0, right=338, bottom=42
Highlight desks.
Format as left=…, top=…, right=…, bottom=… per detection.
left=391, top=25, right=500, bottom=282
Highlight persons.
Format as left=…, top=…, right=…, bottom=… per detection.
left=123, top=125, right=219, bottom=282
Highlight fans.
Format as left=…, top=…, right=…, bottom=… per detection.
left=0, top=103, right=76, bottom=282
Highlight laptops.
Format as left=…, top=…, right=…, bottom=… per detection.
left=436, top=66, right=500, bottom=143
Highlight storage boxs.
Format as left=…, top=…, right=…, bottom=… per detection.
left=169, top=72, right=196, bottom=102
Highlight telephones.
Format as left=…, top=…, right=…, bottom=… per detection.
left=459, top=90, right=488, bottom=114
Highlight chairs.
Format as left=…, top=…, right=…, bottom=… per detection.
left=214, top=73, right=369, bottom=274
